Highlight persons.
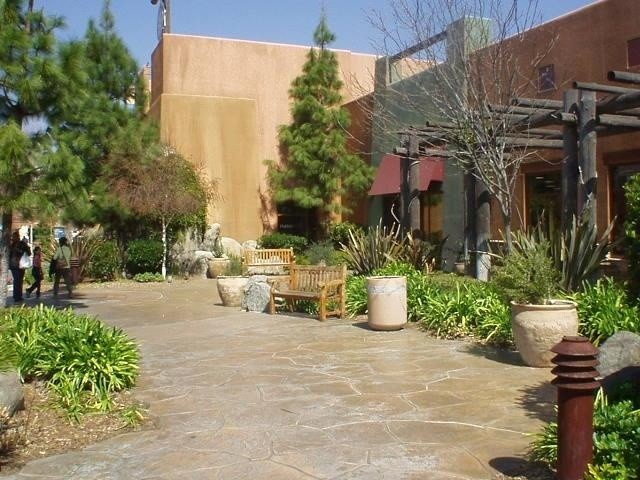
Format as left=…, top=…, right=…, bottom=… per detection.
left=26, top=246, right=45, bottom=298
left=53, top=237, right=73, bottom=298
left=10, top=232, right=31, bottom=302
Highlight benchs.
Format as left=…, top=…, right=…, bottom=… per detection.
left=240, top=246, right=296, bottom=274
left=267, top=264, right=346, bottom=321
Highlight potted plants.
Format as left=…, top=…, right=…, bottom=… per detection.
left=454, top=253, right=471, bottom=273
left=207, top=237, right=226, bottom=279
left=489, top=230, right=579, bottom=368
left=216, top=254, right=249, bottom=307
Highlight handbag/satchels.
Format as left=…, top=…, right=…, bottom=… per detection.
left=56, top=257, right=67, bottom=267
left=19, top=252, right=33, bottom=269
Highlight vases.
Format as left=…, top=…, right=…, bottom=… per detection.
left=366, top=275, right=407, bottom=331
